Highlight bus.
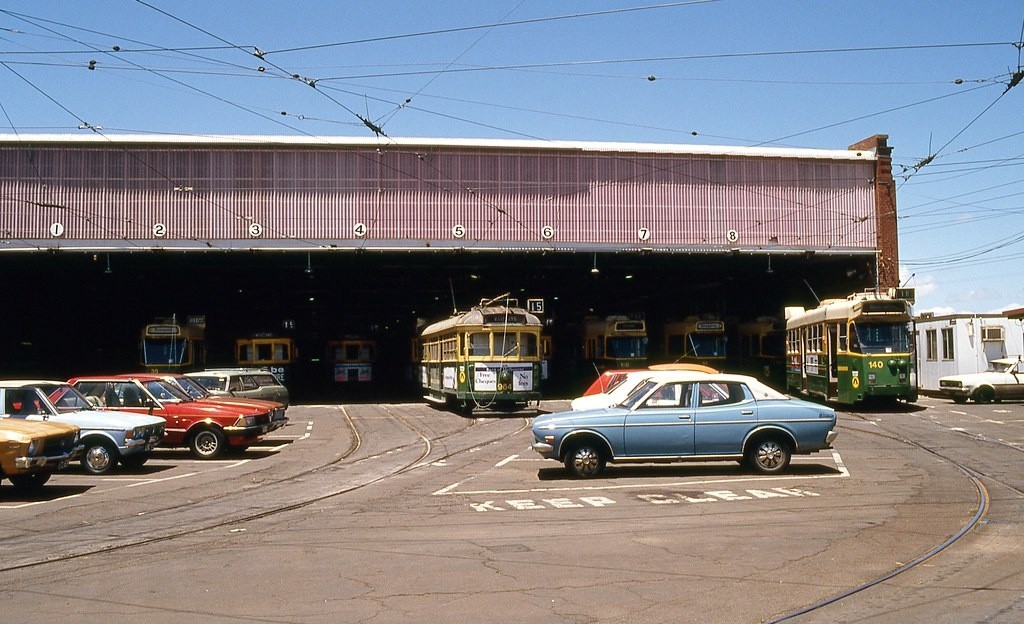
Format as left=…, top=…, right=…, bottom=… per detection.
left=738, top=316, right=785, bottom=380
left=662, top=316, right=728, bottom=375
left=584, top=314, right=649, bottom=376
left=327, top=335, right=376, bottom=398
left=786, top=292, right=914, bottom=410
left=236, top=332, right=299, bottom=401
left=139, top=314, right=207, bottom=376
left=419, top=305, right=544, bottom=417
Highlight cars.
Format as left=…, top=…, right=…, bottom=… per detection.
left=530, top=372, right=839, bottom=480
left=0, top=416, right=81, bottom=492
left=11, top=369, right=291, bottom=461
left=569, top=362, right=731, bottom=410
left=0, top=380, right=168, bottom=476
left=939, top=357, right=1024, bottom=405
